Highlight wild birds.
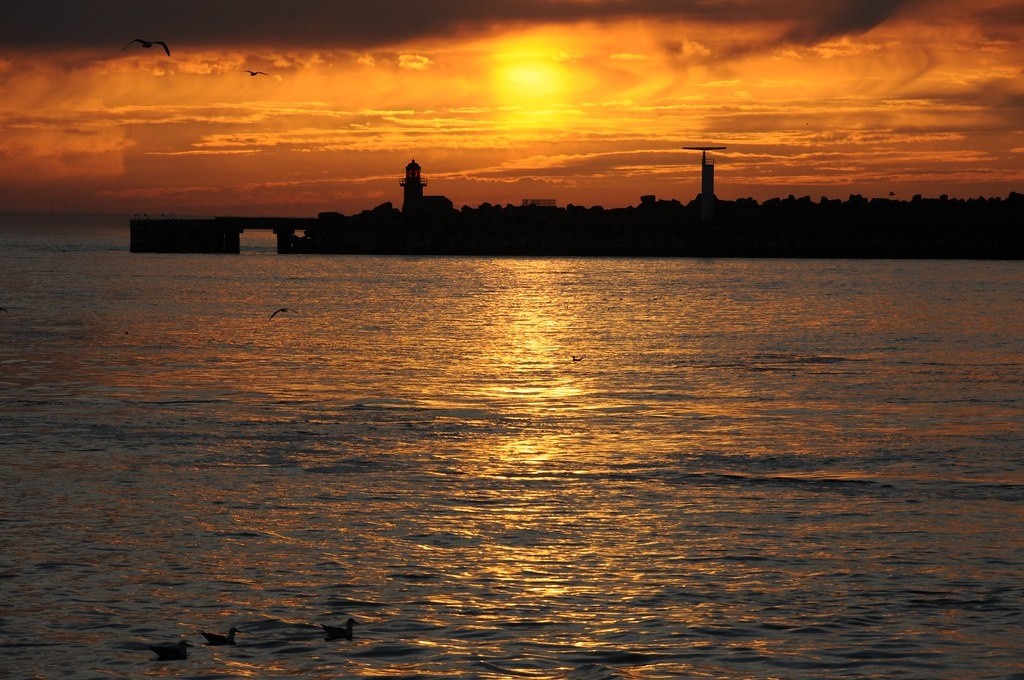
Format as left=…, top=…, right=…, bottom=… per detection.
left=196, top=626, right=242, bottom=646
left=120, top=37, right=172, bottom=57
left=243, top=70, right=268, bottom=77
left=269, top=307, right=300, bottom=320
left=144, top=639, right=193, bottom=661
left=321, top=617, right=361, bottom=642
left=572, top=355, right=583, bottom=362
